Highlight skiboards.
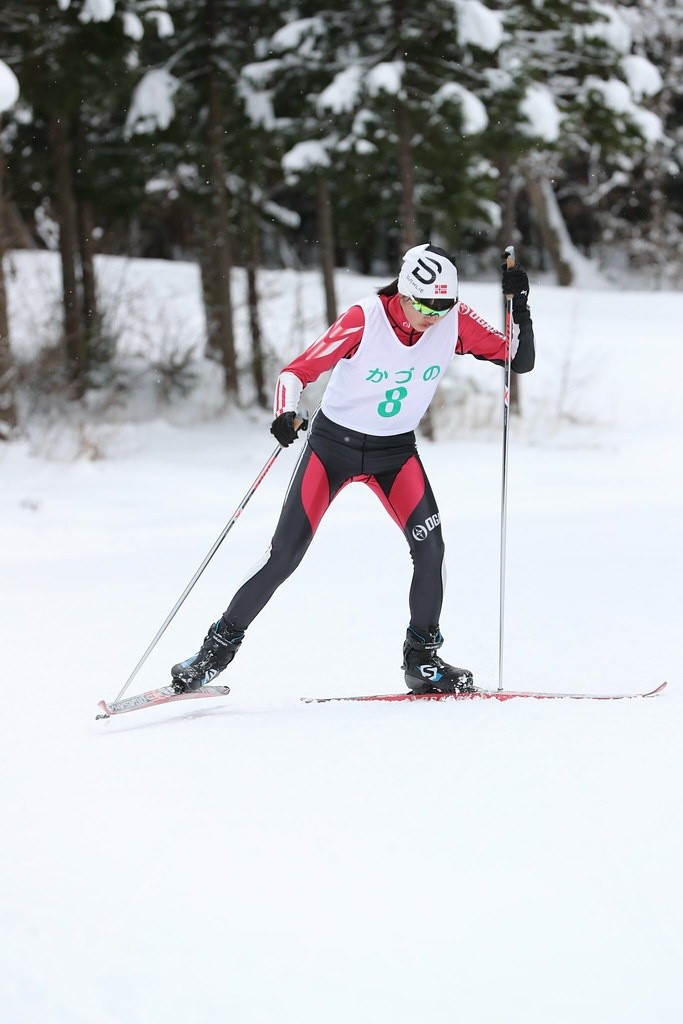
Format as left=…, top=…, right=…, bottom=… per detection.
left=307, top=681, right=668, bottom=703
left=98, top=684, right=232, bottom=718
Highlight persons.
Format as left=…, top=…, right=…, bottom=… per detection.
left=172, top=243, right=536, bottom=693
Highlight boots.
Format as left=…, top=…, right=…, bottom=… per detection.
left=170, top=619, right=245, bottom=694
left=401, top=627, right=473, bottom=692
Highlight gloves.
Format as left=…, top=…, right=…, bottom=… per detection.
left=501, top=252, right=530, bottom=312
left=270, top=411, right=309, bottom=447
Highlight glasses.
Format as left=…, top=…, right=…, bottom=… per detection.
left=409, top=295, right=455, bottom=319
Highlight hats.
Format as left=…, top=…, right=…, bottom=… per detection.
left=398, top=242, right=459, bottom=301
